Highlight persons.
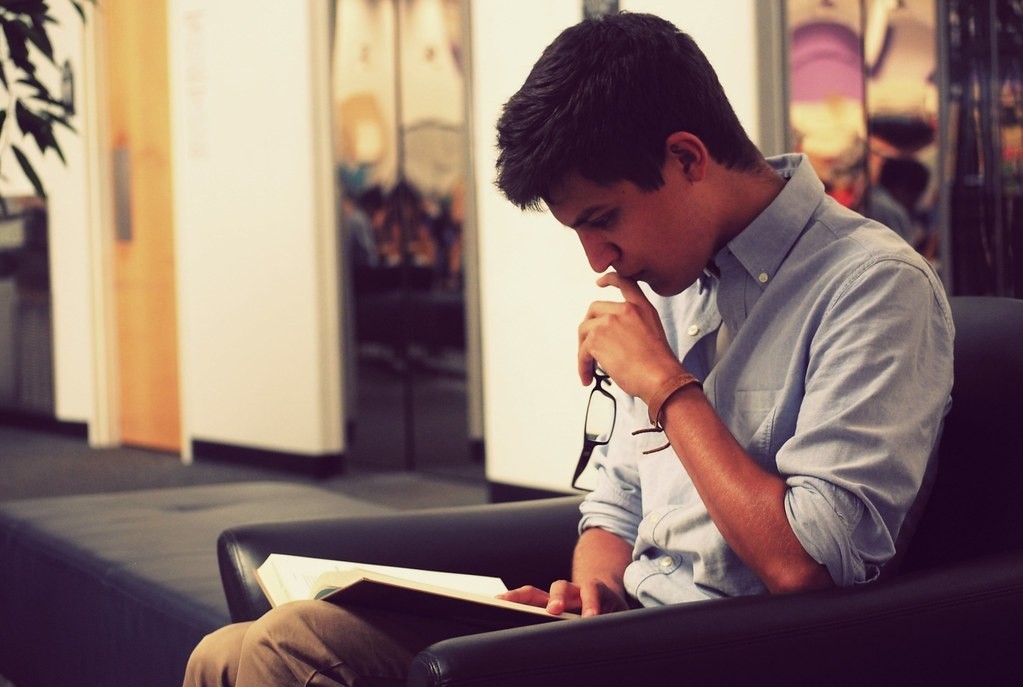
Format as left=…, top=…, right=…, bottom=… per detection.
left=859, top=155, right=928, bottom=251
left=347, top=186, right=385, bottom=264
left=182, top=15, right=958, bottom=687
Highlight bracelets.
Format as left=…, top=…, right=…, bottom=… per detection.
left=648, top=371, right=704, bottom=430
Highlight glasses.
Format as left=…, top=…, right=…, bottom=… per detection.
left=571, top=359, right=617, bottom=493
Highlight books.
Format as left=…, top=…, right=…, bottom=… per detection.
left=253, top=553, right=581, bottom=653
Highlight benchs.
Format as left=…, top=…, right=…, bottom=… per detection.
left=0, top=478, right=395, bottom=687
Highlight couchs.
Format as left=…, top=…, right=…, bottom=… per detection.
left=217, top=296, right=1023, bottom=687
